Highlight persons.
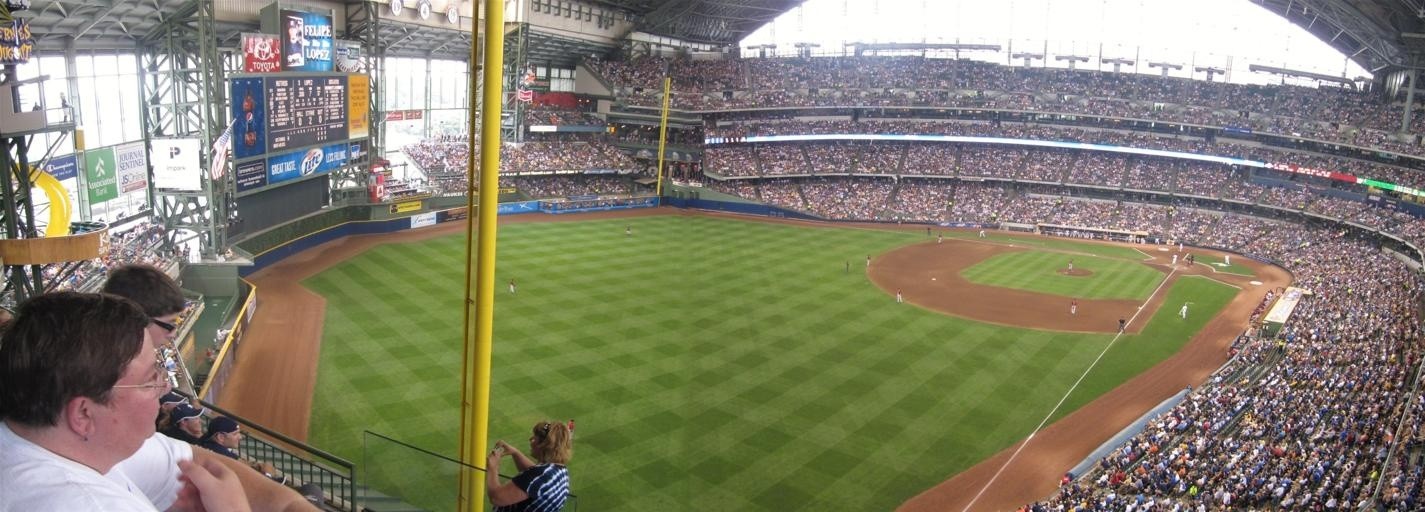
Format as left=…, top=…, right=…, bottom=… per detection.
left=383, top=48, right=1425, bottom=512
left=284, top=19, right=302, bottom=65
left=1, top=215, right=327, bottom=511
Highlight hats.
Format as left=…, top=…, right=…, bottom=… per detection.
left=161, top=393, right=186, bottom=404
left=201, top=416, right=239, bottom=440
left=170, top=404, right=205, bottom=424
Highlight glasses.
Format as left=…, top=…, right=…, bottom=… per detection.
left=152, top=317, right=180, bottom=335
left=113, top=364, right=167, bottom=391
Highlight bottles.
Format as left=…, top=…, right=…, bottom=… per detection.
left=242, top=88, right=257, bottom=146
